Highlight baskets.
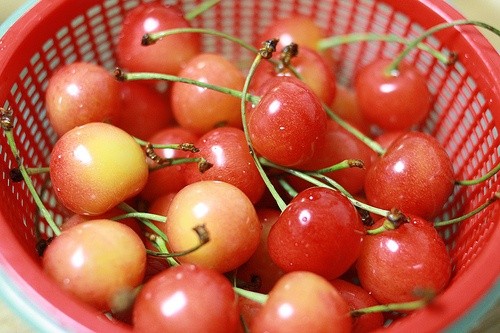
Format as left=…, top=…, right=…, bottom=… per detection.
left=0, top=1, right=500, bottom=333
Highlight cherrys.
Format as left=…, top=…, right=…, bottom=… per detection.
left=0, top=0, right=500, bottom=333
